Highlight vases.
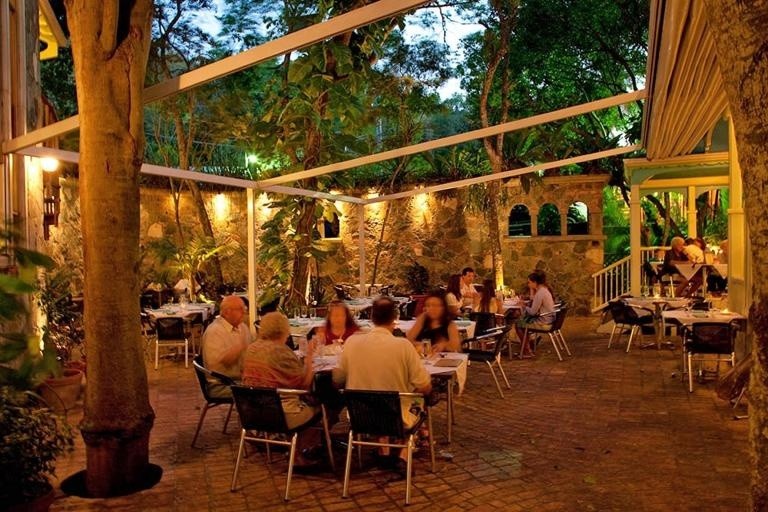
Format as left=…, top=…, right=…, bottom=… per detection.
left=1, top=481, right=55, bottom=512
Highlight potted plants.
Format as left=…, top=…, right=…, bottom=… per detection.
left=37, top=277, right=83, bottom=412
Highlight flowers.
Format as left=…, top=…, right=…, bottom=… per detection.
left=0, top=385, right=80, bottom=502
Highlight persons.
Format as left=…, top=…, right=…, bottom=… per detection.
left=389, top=319, right=454, bottom=461
left=201, top=294, right=254, bottom=402
left=302, top=300, right=362, bottom=460
left=640, top=228, right=728, bottom=298
left=405, top=292, right=460, bottom=437
left=443, top=267, right=555, bottom=359
left=240, top=311, right=327, bottom=470
left=331, top=297, right=433, bottom=479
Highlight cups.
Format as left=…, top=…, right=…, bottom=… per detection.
left=296, top=335, right=343, bottom=371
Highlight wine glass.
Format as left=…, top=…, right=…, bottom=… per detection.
left=354, top=306, right=360, bottom=322
left=289, top=305, right=322, bottom=328
left=643, top=284, right=728, bottom=319
left=421, top=338, right=433, bottom=366
left=166, top=293, right=196, bottom=315
left=495, top=285, right=516, bottom=301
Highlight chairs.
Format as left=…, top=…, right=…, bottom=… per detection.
left=607, top=252, right=739, bottom=394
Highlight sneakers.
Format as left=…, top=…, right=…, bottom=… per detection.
left=376, top=454, right=393, bottom=471
left=388, top=456, right=416, bottom=478
left=517, top=351, right=537, bottom=359
